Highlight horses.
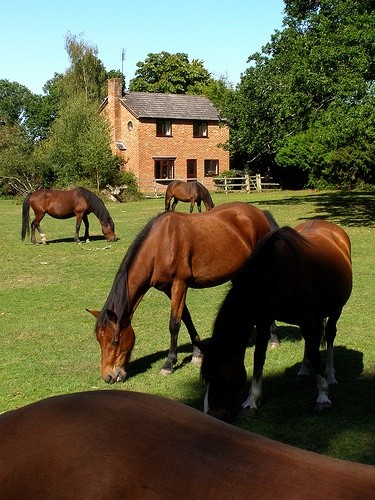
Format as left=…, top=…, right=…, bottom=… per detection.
left=0, top=389, right=375, bottom=500
left=86, top=201, right=284, bottom=385
left=21, top=187, right=115, bottom=246
left=199, top=218, right=353, bottom=422
left=165, top=180, right=214, bottom=214
